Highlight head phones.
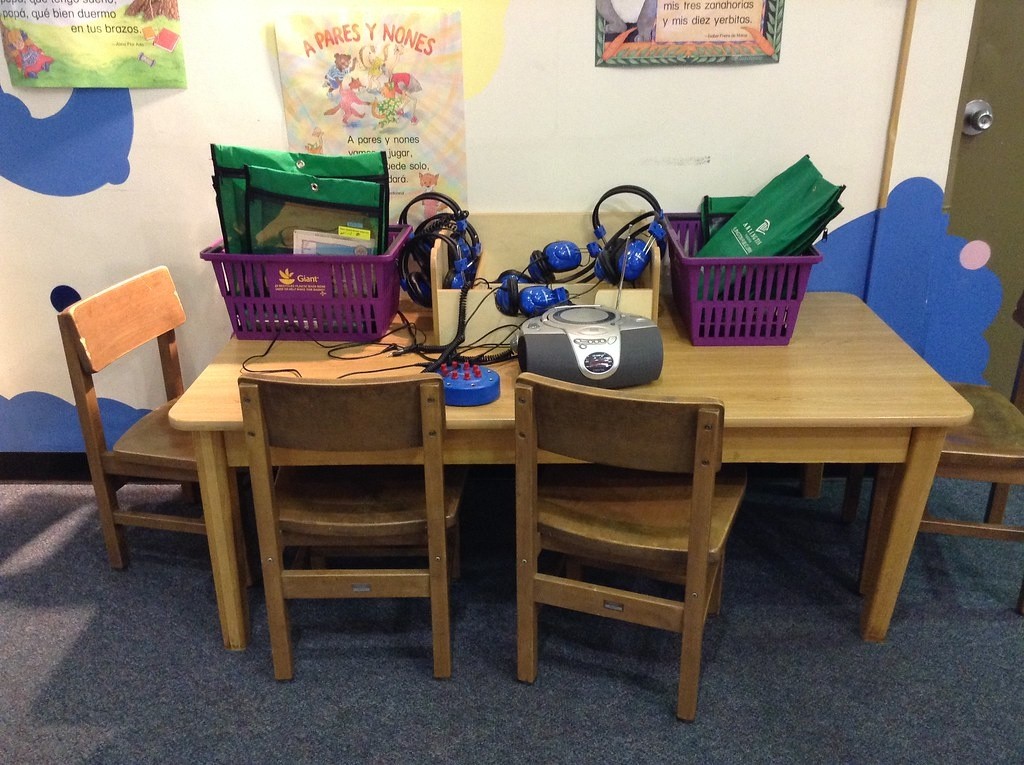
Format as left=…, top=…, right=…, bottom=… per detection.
left=396, top=191, right=482, bottom=309
left=495, top=185, right=668, bottom=318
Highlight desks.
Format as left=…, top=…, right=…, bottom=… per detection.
left=167, top=292, right=975, bottom=653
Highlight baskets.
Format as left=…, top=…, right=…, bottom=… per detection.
left=200, top=225, right=413, bottom=341
left=662, top=212, right=823, bottom=346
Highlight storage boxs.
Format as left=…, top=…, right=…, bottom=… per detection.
left=429, top=210, right=660, bottom=350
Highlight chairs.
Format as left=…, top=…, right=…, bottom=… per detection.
left=842, top=286, right=1024, bottom=619
left=57, top=265, right=256, bottom=589
left=514, top=372, right=749, bottom=723
left=237, top=373, right=467, bottom=681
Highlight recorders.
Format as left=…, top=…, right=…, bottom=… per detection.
left=517, top=224, right=664, bottom=389
